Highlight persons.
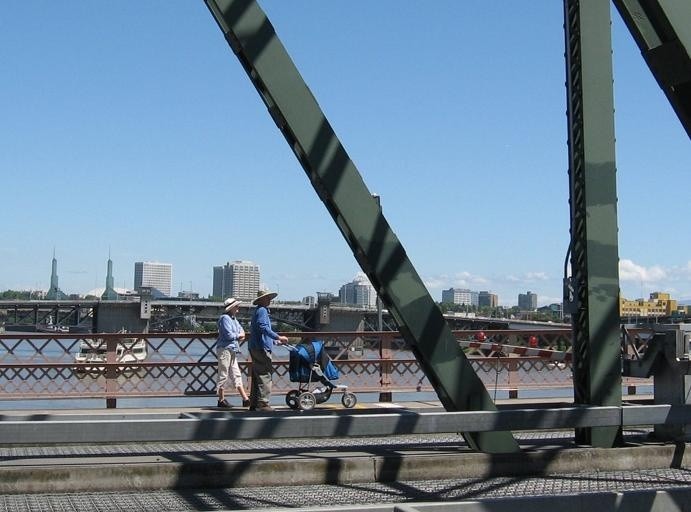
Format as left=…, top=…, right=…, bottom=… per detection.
left=216, top=298, right=251, bottom=408
left=247, top=288, right=288, bottom=412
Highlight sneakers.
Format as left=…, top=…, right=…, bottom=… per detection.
left=243, top=399, right=276, bottom=411
left=218, top=399, right=233, bottom=407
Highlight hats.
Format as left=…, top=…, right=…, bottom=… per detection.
left=224, top=297, right=242, bottom=313
left=253, top=290, right=277, bottom=305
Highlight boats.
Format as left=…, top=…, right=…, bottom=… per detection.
left=71, top=325, right=148, bottom=372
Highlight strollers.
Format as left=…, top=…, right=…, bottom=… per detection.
left=273, top=337, right=358, bottom=411
